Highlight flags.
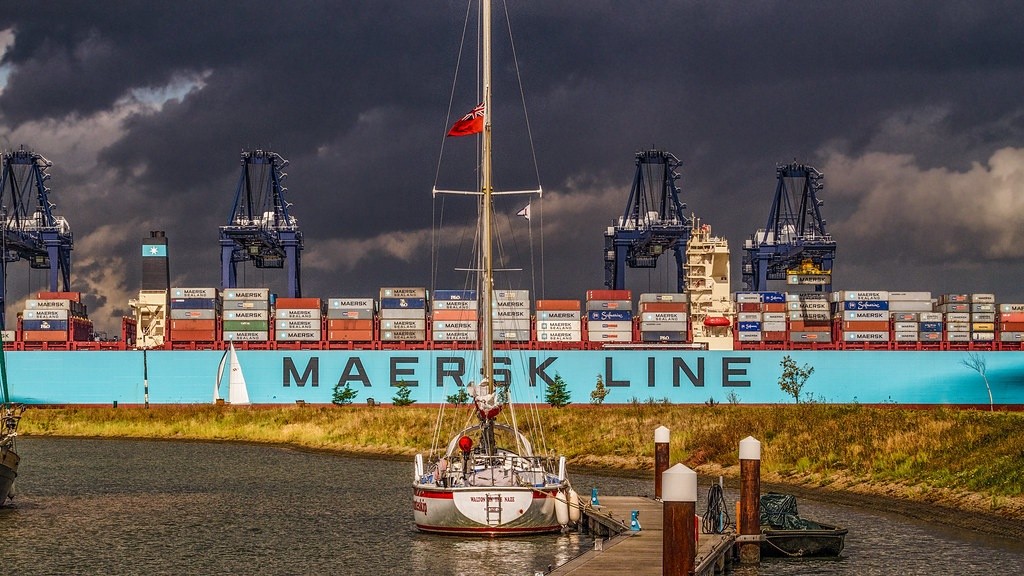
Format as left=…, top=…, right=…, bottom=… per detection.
left=447, top=101, right=484, bottom=137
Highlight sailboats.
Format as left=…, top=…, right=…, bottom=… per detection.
left=410, top=1, right=578, bottom=539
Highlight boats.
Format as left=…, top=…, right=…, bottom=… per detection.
left=1, top=413, right=24, bottom=507
left=714, top=518, right=851, bottom=557
